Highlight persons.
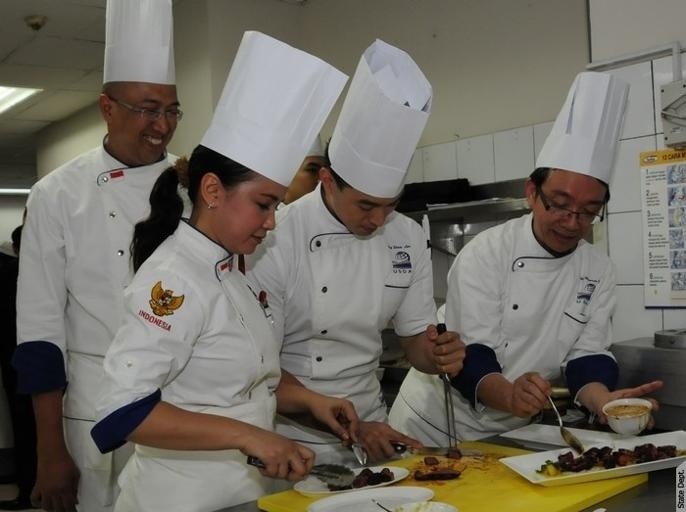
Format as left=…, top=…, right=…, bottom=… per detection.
left=88, top=141, right=361, bottom=512
left=244, top=135, right=474, bottom=495
left=13, top=83, right=199, bottom=512
left=386, top=166, right=670, bottom=450
left=274, top=153, right=329, bottom=208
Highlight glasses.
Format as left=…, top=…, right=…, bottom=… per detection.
left=536, top=185, right=605, bottom=225
left=108, top=95, right=183, bottom=122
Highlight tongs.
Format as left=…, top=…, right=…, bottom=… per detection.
left=246, top=456, right=356, bottom=489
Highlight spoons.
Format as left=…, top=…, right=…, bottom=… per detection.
left=340, top=420, right=371, bottom=465
left=543, top=392, right=586, bottom=455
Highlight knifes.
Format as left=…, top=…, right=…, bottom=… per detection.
left=345, top=441, right=483, bottom=457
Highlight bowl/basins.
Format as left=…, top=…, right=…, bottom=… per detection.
left=384, top=501, right=459, bottom=511
left=602, top=396, right=652, bottom=436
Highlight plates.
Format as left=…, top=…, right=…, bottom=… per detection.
left=293, top=466, right=407, bottom=498
left=306, top=486, right=435, bottom=511
left=498, top=422, right=640, bottom=450
left=498, top=442, right=686, bottom=487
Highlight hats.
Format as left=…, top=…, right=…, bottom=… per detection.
left=328, top=38, right=433, bottom=199
left=199, top=30, right=350, bottom=189
left=306, top=132, right=325, bottom=158
left=535, top=71, right=632, bottom=185
left=103, top=0, right=176, bottom=86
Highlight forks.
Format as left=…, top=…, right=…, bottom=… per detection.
left=438, top=324, right=459, bottom=450
